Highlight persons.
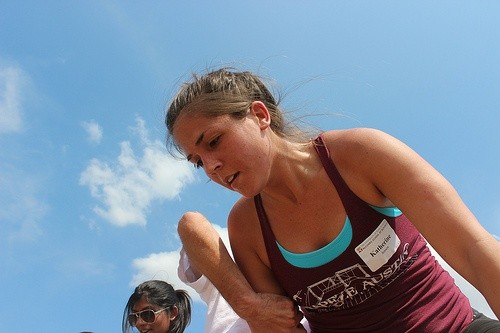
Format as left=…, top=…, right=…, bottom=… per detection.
left=165, top=66, right=500, bottom=333
left=177, top=212, right=312, bottom=333
left=122, top=280, right=192, bottom=333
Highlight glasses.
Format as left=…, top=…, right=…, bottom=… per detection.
left=128, top=307, right=169, bottom=327
